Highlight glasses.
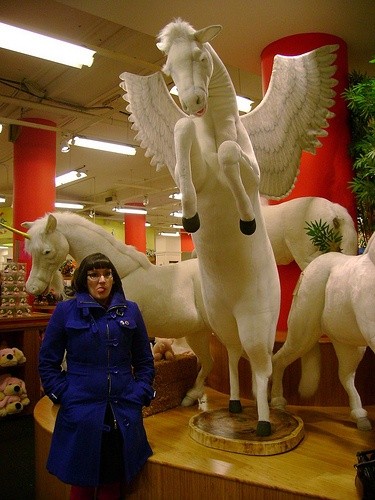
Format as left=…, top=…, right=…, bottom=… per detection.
left=87, top=272, right=113, bottom=280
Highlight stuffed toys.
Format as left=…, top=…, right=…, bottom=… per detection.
left=153, top=339, right=176, bottom=361
left=0, top=344, right=26, bottom=367
left=0, top=374, right=30, bottom=417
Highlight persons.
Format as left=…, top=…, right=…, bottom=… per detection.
left=40, top=252, right=155, bottom=500
left=353, top=346, right=375, bottom=500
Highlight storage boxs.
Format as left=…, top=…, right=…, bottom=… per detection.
left=141, top=353, right=197, bottom=416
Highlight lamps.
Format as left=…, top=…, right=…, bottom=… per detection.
left=112, top=207, right=148, bottom=216
left=55, top=203, right=86, bottom=212
left=0, top=20, right=96, bottom=70
left=158, top=192, right=184, bottom=238
left=55, top=166, right=89, bottom=187
left=169, top=83, right=255, bottom=114
left=68, top=135, right=137, bottom=157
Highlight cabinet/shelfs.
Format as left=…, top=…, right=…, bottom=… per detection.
left=0, top=312, right=53, bottom=493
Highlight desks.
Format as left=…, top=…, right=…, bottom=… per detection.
left=33, top=386, right=375, bottom=500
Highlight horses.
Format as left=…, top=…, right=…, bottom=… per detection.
left=0, top=207, right=213, bottom=408
left=268, top=230, right=375, bottom=430
left=259, top=195, right=360, bottom=275
left=153, top=15, right=282, bottom=439
